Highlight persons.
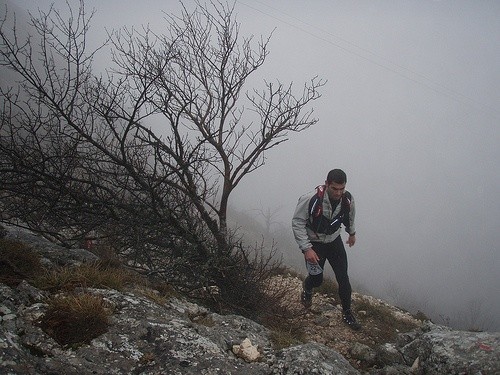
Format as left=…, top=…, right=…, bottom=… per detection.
left=292, top=169, right=361, bottom=331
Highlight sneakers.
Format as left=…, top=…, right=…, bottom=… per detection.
left=301, top=291, right=312, bottom=307
left=342, top=312, right=360, bottom=330
